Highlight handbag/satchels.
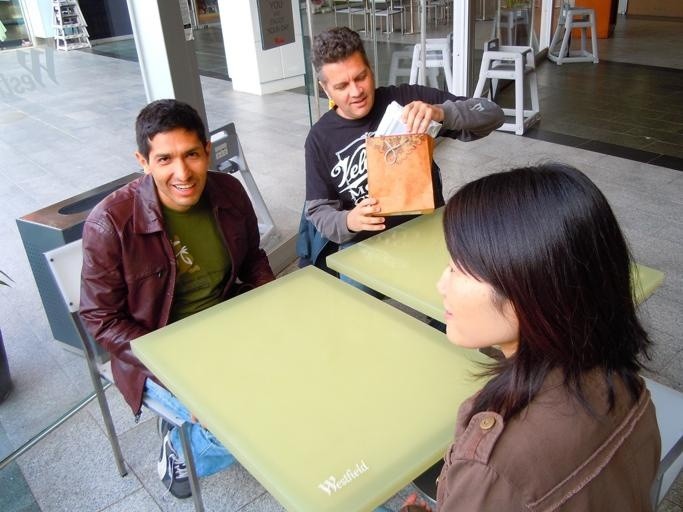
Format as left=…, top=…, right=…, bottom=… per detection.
left=364, top=132, right=436, bottom=218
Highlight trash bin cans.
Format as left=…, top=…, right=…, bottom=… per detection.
left=17, top=172, right=146, bottom=364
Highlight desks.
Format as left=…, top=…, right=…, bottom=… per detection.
left=326, top=198, right=664, bottom=372
left=129, top=263, right=511, bottom=512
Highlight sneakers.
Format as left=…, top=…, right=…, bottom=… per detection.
left=157, top=416, right=193, bottom=497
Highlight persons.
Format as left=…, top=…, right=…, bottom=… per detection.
left=79, top=99, right=276, bottom=499
left=399, top=162, right=660, bottom=510
left=306, top=26, right=505, bottom=300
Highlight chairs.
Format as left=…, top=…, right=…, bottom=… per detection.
left=631, top=371, right=681, bottom=512
left=40, top=238, right=205, bottom=511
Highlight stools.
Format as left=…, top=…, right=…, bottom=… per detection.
left=51, top=0, right=93, bottom=54
left=328, top=1, right=599, bottom=136
